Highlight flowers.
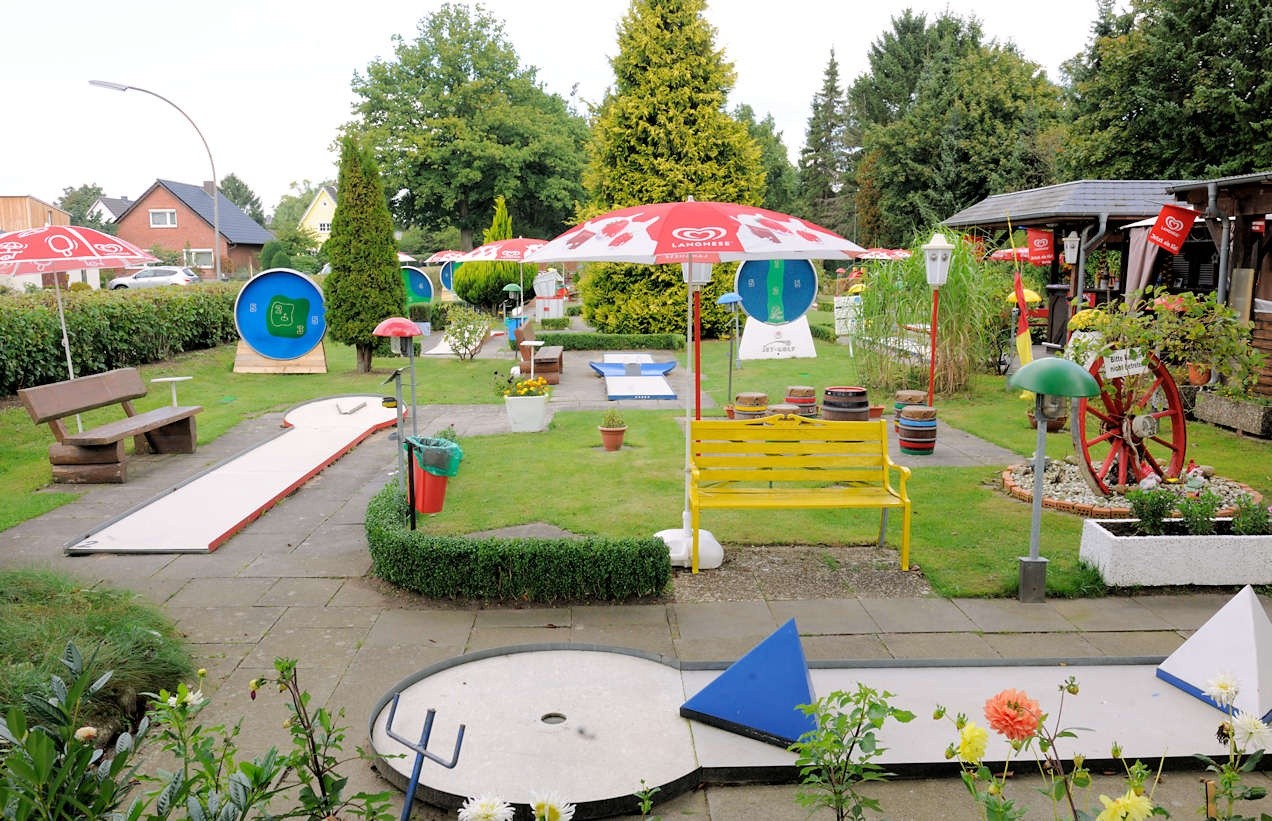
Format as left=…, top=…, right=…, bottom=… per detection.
left=1019, top=390, right=1035, bottom=414
left=1205, top=383, right=1270, bottom=405
left=488, top=370, right=556, bottom=397
left=1153, top=365, right=1191, bottom=386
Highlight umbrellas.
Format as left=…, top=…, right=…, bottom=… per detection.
left=455, top=235, right=549, bottom=327
left=516, top=195, right=869, bottom=569
left=0, top=210, right=165, bottom=433
left=423, top=249, right=469, bottom=290
left=857, top=246, right=913, bottom=260
left=397, top=252, right=417, bottom=262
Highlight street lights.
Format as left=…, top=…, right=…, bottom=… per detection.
left=87, top=78, right=222, bottom=288
left=831, top=182, right=859, bottom=263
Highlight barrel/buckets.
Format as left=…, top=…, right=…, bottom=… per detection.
left=894, top=390, right=929, bottom=431
left=822, top=386, right=871, bottom=421
left=898, top=406, right=936, bottom=456
left=786, top=386, right=817, bottom=419
left=735, top=393, right=769, bottom=419
left=765, top=404, right=801, bottom=425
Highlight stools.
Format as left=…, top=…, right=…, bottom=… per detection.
left=893, top=390, right=938, bottom=455
left=734, top=385, right=818, bottom=455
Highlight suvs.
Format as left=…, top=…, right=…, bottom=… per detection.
left=109, top=265, right=200, bottom=290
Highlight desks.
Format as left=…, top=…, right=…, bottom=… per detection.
left=150, top=377, right=193, bottom=406
left=519, top=339, right=545, bottom=381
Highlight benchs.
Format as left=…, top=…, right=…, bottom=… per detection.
left=515, top=321, right=564, bottom=384
left=17, top=366, right=204, bottom=484
left=688, top=414, right=912, bottom=575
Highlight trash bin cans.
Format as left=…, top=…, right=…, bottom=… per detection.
left=406, top=436, right=458, bottom=513
left=509, top=316, right=527, bottom=340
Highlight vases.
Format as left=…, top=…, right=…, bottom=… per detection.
left=1027, top=414, right=1068, bottom=432
left=1193, top=390, right=1272, bottom=439
left=1152, top=379, right=1194, bottom=414
left=504, top=394, right=549, bottom=433
left=1186, top=361, right=1212, bottom=386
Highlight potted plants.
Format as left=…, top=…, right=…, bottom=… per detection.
left=1079, top=486, right=1272, bottom=586
left=724, top=400, right=736, bottom=419
left=597, top=409, right=629, bottom=451
left=869, top=399, right=885, bottom=416
